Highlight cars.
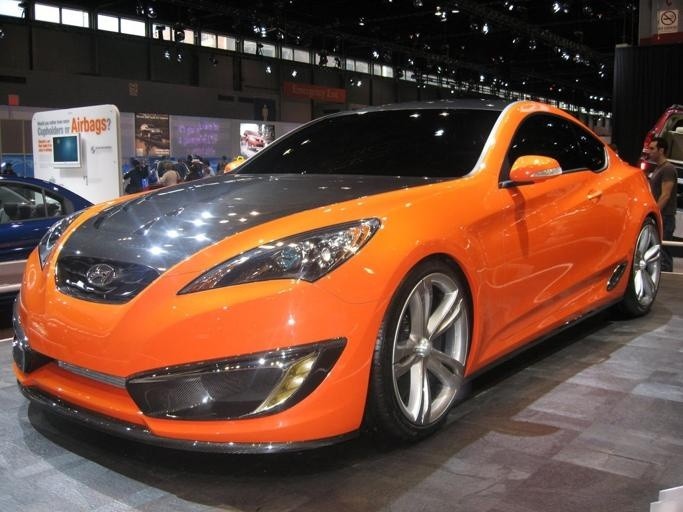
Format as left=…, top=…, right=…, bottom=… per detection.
left=0, top=177, right=94, bottom=296
left=636, top=105, right=683, bottom=199
left=140, top=123, right=163, bottom=137
left=11, top=100, right=663, bottom=456
left=244, top=130, right=266, bottom=146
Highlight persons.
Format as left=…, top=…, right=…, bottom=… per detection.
left=641, top=137, right=679, bottom=272
left=2, top=162, right=17, bottom=177
left=124, top=154, right=229, bottom=193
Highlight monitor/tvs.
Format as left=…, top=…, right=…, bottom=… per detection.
left=51, top=132, right=82, bottom=168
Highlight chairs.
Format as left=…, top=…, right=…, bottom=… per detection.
left=4, top=203, right=60, bottom=221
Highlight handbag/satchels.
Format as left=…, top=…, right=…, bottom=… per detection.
left=124, top=183, right=136, bottom=194
